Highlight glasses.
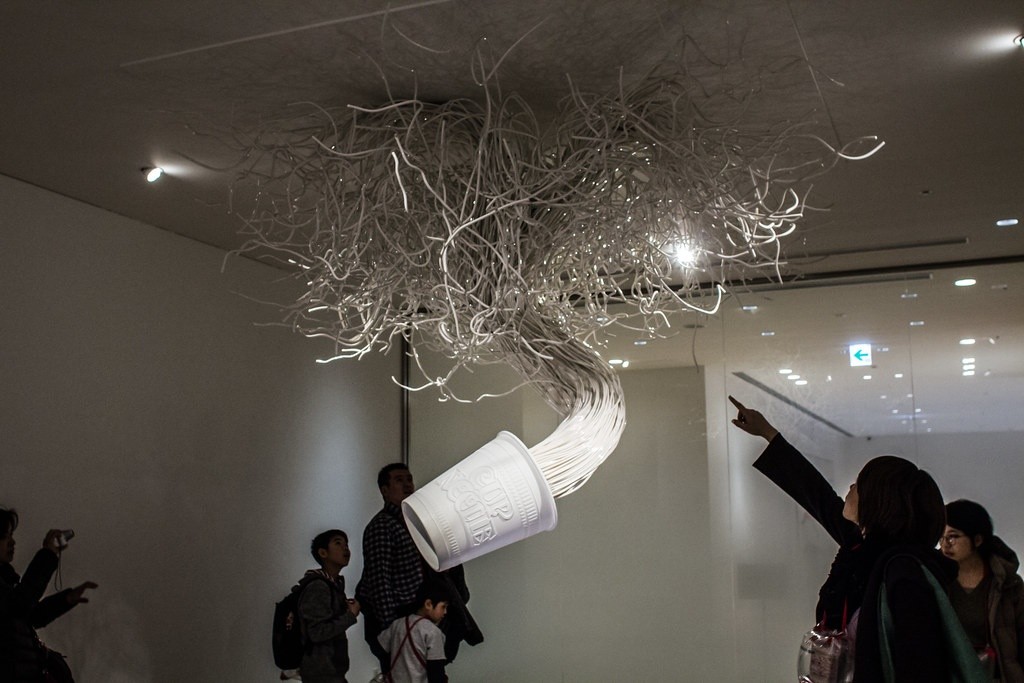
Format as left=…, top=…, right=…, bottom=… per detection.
left=939, top=534, right=967, bottom=547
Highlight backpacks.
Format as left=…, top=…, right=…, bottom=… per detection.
left=272, top=569, right=334, bottom=670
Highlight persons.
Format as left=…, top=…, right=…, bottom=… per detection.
left=939, top=499, right=1024, bottom=683
left=355, top=462, right=442, bottom=682
left=728, top=395, right=992, bottom=682
left=370, top=586, right=449, bottom=683
left=0, top=507, right=99, bottom=683
left=295, top=529, right=360, bottom=683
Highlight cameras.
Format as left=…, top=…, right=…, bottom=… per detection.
left=58, top=529, right=75, bottom=547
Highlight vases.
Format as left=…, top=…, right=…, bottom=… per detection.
left=400, top=429, right=558, bottom=572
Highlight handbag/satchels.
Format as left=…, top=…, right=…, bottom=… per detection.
left=797, top=621, right=849, bottom=683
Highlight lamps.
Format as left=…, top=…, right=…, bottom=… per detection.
left=141, top=166, right=162, bottom=183
